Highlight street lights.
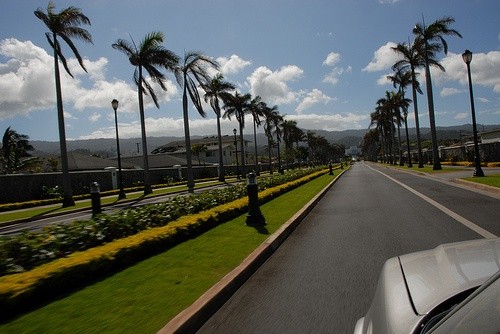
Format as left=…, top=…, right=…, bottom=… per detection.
left=111, top=99, right=127, bottom=199
left=403, top=111, right=413, bottom=168
left=462, top=49, right=486, bottom=178
left=233, top=128, right=240, bottom=179
